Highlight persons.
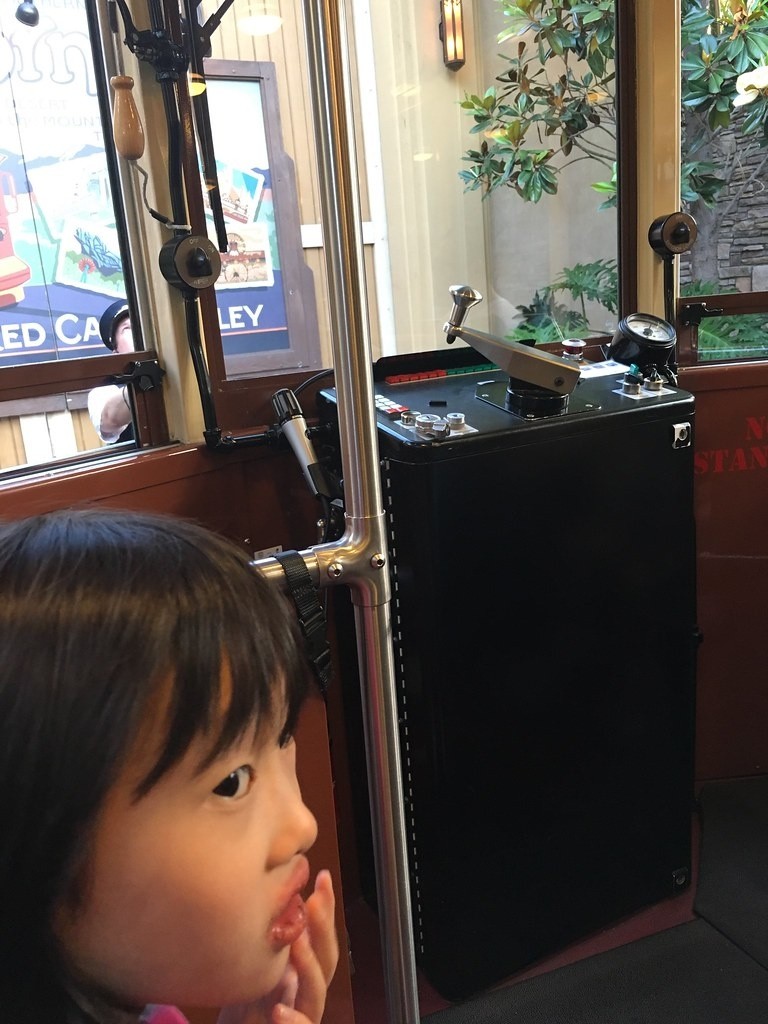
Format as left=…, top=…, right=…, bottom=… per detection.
left=87, top=300, right=135, bottom=444
left=0, top=510, right=340, bottom=1023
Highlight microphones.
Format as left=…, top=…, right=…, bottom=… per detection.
left=270, top=388, right=326, bottom=500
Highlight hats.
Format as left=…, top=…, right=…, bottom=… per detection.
left=99, top=299, right=130, bottom=351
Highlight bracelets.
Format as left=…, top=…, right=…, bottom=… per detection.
left=123, top=387, right=132, bottom=412
